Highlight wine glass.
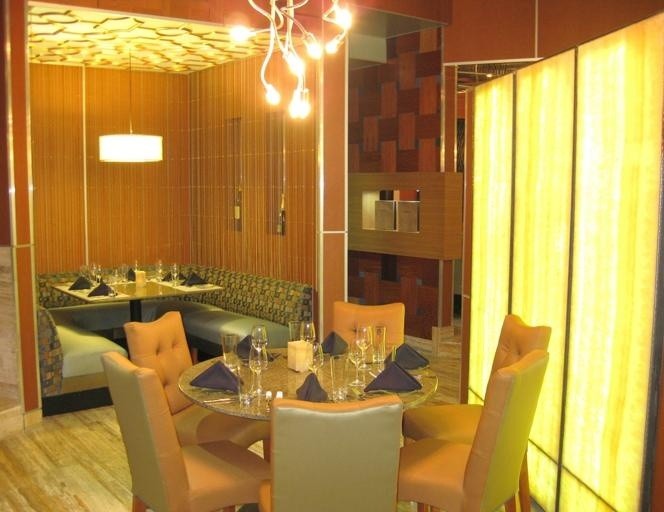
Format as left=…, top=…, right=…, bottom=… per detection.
left=77, top=259, right=181, bottom=296
left=219, top=321, right=386, bottom=409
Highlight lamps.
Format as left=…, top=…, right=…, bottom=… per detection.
left=98, top=50, right=163, bottom=165
left=230, top=0, right=356, bottom=121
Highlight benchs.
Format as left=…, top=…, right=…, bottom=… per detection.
left=36, top=263, right=314, bottom=365
left=37, top=304, right=130, bottom=417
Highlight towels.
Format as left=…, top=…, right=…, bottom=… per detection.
left=294, top=332, right=431, bottom=403
left=235, top=334, right=272, bottom=362
left=189, top=360, right=245, bottom=394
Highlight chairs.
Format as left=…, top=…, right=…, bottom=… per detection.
left=122, top=310, right=271, bottom=464
left=100, top=351, right=271, bottom=512
left=397, top=349, right=550, bottom=512
left=331, top=300, right=406, bottom=354
left=260, top=394, right=405, bottom=512
left=401, top=313, right=553, bottom=512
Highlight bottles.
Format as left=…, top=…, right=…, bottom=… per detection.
left=274, top=192, right=286, bottom=235
left=232, top=187, right=243, bottom=231
